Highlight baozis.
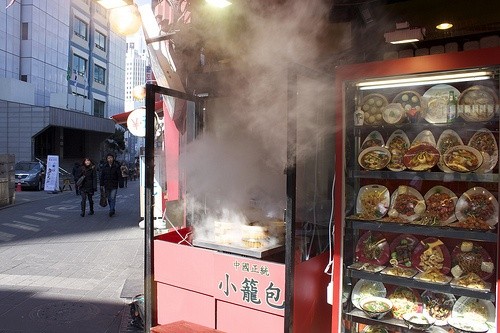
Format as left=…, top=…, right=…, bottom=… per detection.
left=481, top=261, right=494, bottom=273
left=461, top=242, right=473, bottom=252
left=414, top=203, right=426, bottom=213
left=451, top=265, right=463, bottom=278
left=398, top=186, right=408, bottom=195
left=361, top=94, right=420, bottom=123
left=388, top=209, right=399, bottom=218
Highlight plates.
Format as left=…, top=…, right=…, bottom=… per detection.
left=348, top=85, right=500, bottom=326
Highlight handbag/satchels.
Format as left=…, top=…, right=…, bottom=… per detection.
left=99, top=187, right=108, bottom=207
left=124, top=169, right=130, bottom=174
left=76, top=175, right=85, bottom=187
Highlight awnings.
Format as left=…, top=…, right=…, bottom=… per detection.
left=112, top=101, right=163, bottom=129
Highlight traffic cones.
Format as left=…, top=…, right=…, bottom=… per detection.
left=15, top=178, right=21, bottom=192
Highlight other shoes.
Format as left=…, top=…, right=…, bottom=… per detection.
left=109, top=209, right=115, bottom=217
left=80, top=213, right=85, bottom=217
left=88, top=211, right=94, bottom=215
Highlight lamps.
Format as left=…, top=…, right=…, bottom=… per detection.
left=383, top=21, right=427, bottom=45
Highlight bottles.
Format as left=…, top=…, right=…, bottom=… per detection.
left=446, top=91, right=457, bottom=125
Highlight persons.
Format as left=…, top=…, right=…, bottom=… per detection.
left=119, top=157, right=137, bottom=188
left=100, top=153, right=124, bottom=216
left=73, top=160, right=83, bottom=195
left=78, top=156, right=97, bottom=217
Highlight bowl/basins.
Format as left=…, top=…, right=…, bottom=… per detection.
left=359, top=297, right=393, bottom=320
left=443, top=145, right=484, bottom=173
left=402, top=144, right=440, bottom=171
left=358, top=146, right=391, bottom=170
left=447, top=318, right=489, bottom=333
left=402, top=313, right=436, bottom=331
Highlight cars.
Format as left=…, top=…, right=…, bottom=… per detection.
left=13, top=160, right=47, bottom=190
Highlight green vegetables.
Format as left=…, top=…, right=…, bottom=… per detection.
left=364, top=288, right=453, bottom=324
left=365, top=327, right=387, bottom=333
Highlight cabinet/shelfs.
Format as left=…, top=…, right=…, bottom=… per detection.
left=331, top=65, right=500, bottom=333
